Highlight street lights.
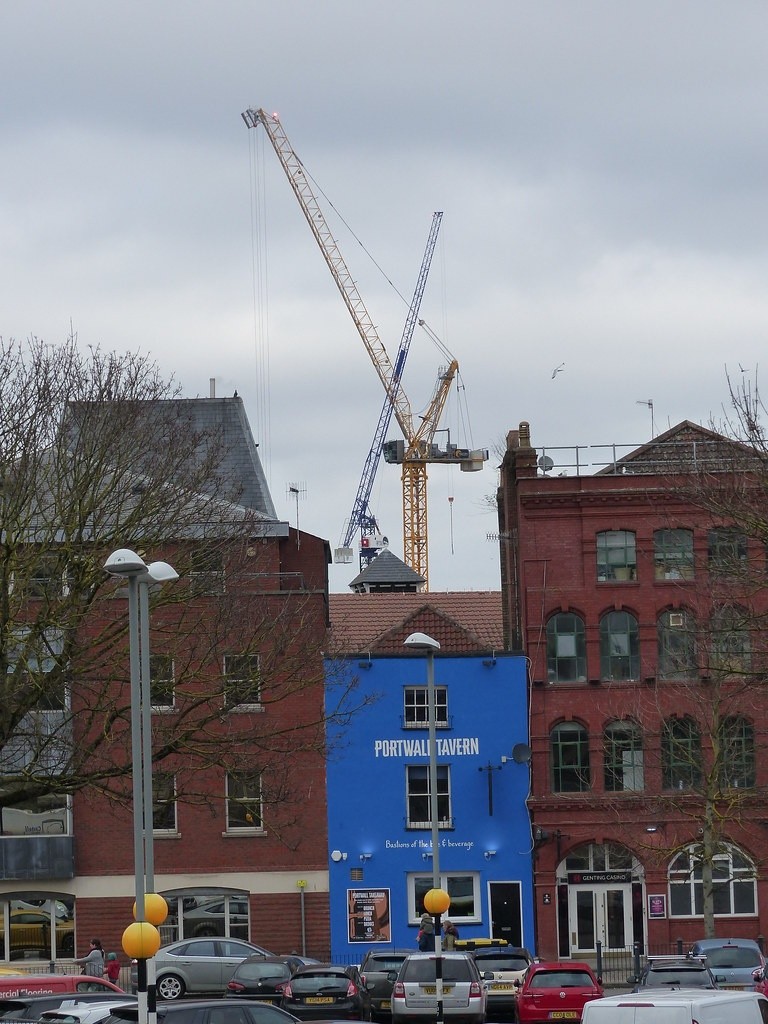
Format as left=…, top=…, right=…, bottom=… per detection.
left=138, top=561, right=181, bottom=891
left=403, top=632, right=440, bottom=890
left=103, top=549, right=150, bottom=920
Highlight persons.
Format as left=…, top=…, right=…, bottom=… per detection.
left=415, top=912, right=435, bottom=952
left=441, top=919, right=459, bottom=951
left=103, top=952, right=120, bottom=985
left=72, top=939, right=105, bottom=992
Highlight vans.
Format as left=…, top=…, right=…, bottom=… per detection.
left=579, top=938, right=768, bottom=1024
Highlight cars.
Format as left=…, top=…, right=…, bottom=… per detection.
left=0, top=897, right=74, bottom=960
left=0, top=966, right=304, bottom=1024
left=130, top=936, right=324, bottom=1000
left=162, top=893, right=198, bottom=917
left=514, top=961, right=605, bottom=1024
left=172, top=896, right=249, bottom=941
left=454, top=939, right=545, bottom=1015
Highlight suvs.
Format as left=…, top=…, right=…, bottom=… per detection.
left=279, top=965, right=376, bottom=1021
left=356, top=946, right=424, bottom=1016
left=223, top=955, right=306, bottom=1007
left=387, top=950, right=494, bottom=1024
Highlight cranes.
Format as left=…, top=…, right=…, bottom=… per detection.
left=333, top=211, right=445, bottom=574
left=244, top=105, right=490, bottom=593
